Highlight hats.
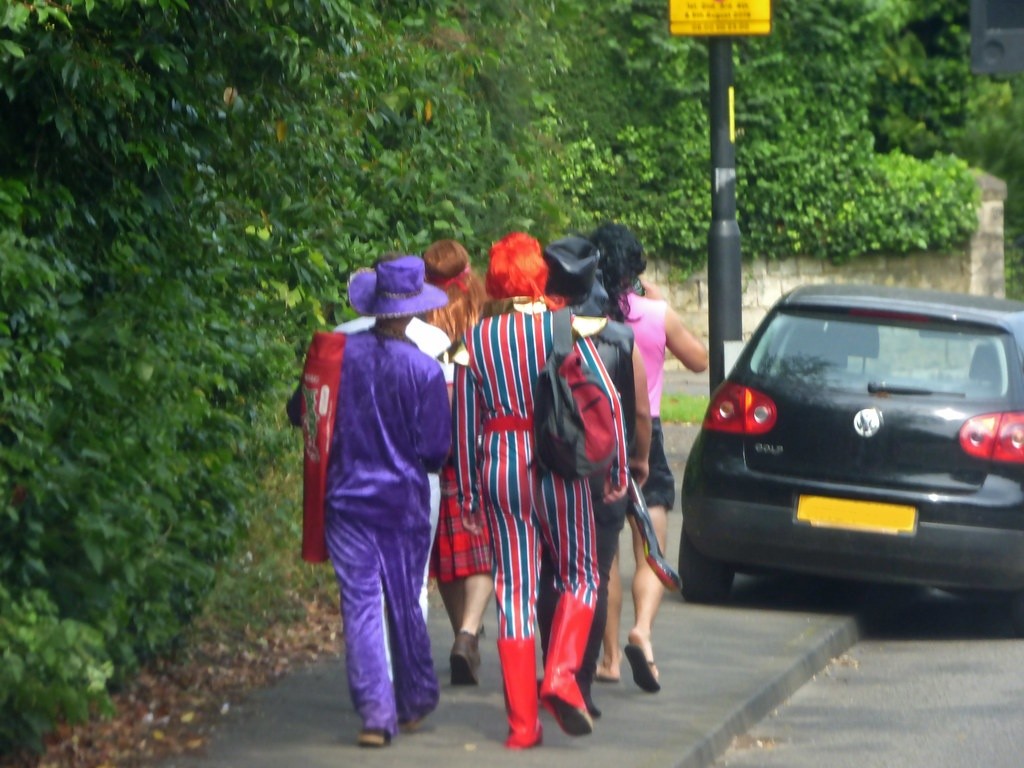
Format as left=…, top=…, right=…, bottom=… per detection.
left=346, top=256, right=450, bottom=318
left=541, top=237, right=609, bottom=317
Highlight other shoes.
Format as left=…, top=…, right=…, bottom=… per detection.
left=400, top=712, right=429, bottom=734
left=449, top=628, right=483, bottom=685
left=551, top=693, right=594, bottom=736
left=358, top=728, right=387, bottom=745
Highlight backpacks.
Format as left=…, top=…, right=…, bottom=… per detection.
left=532, top=308, right=619, bottom=480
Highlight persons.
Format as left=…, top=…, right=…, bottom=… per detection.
left=418, top=240, right=494, bottom=684
left=328, top=257, right=455, bottom=685
left=537, top=222, right=707, bottom=716
left=451, top=233, right=629, bottom=750
left=286, top=256, right=451, bottom=745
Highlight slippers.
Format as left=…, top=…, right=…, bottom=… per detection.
left=595, top=668, right=620, bottom=684
left=623, top=645, right=663, bottom=693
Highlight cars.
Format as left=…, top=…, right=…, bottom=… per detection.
left=677, top=283, right=1024, bottom=639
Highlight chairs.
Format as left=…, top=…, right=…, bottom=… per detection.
left=826, top=321, right=880, bottom=374
